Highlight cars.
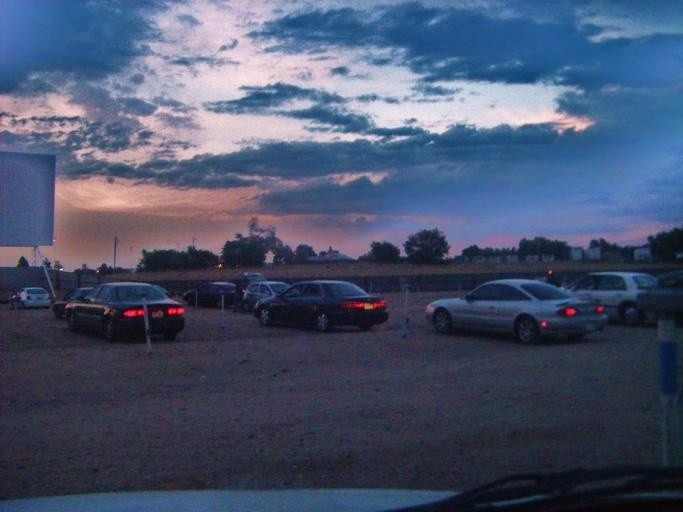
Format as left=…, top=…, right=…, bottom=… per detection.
left=51, top=282, right=185, bottom=343
left=424, top=281, right=608, bottom=343
left=637, top=271, right=682, bottom=330
left=564, top=271, right=660, bottom=326
left=252, top=281, right=389, bottom=332
left=9, top=288, right=51, bottom=310
left=184, top=273, right=299, bottom=312
